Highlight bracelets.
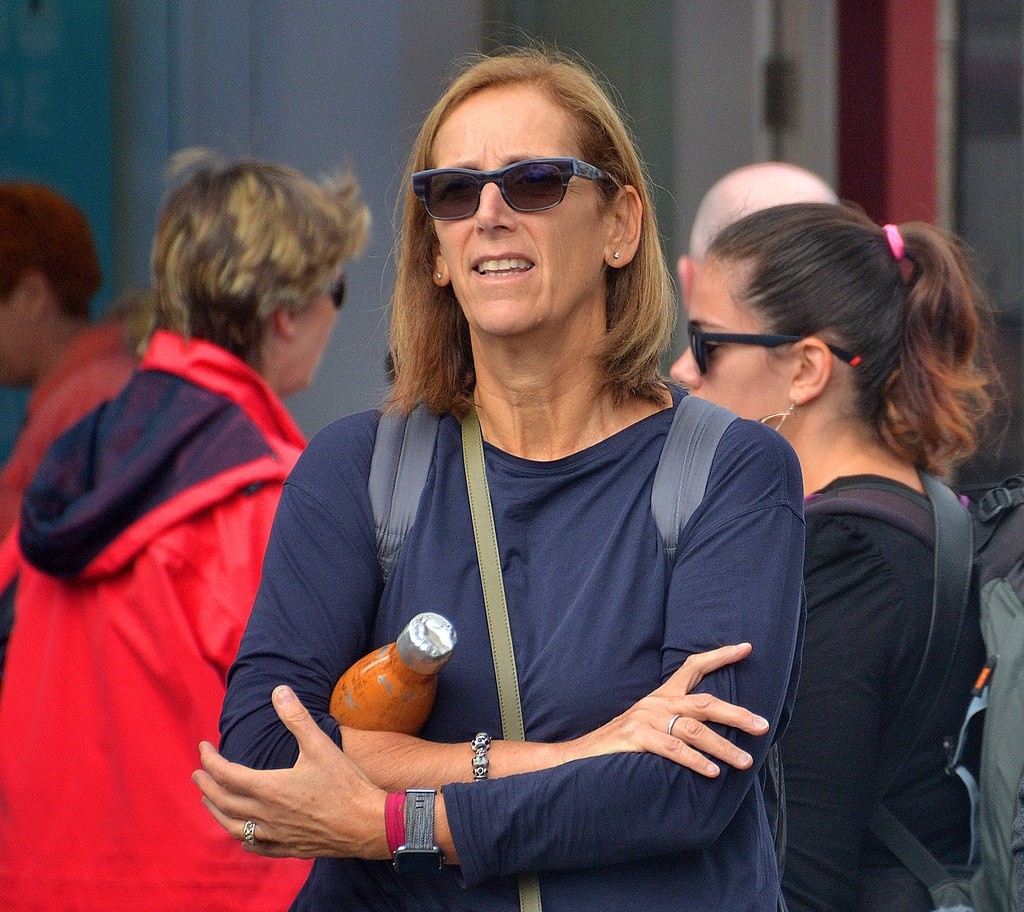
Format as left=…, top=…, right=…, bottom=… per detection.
left=385, top=791, right=405, bottom=855
left=470, top=731, right=489, bottom=783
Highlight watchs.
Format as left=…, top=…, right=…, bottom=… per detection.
left=391, top=788, right=445, bottom=882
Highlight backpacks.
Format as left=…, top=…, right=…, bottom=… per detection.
left=863, top=474, right=1024, bottom=912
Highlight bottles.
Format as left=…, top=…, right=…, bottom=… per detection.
left=329, top=611, right=457, bottom=737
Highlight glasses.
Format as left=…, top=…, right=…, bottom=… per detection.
left=410, top=157, right=612, bottom=220
left=688, top=323, right=863, bottom=376
left=329, top=275, right=347, bottom=310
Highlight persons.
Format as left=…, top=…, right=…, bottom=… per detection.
left=0, top=164, right=362, bottom=912
left=0, top=178, right=140, bottom=540
left=192, top=55, right=805, bottom=912
left=679, top=159, right=838, bottom=316
left=674, top=201, right=1024, bottom=912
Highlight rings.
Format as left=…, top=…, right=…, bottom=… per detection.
left=247, top=821, right=254, bottom=845
left=667, top=715, right=681, bottom=735
